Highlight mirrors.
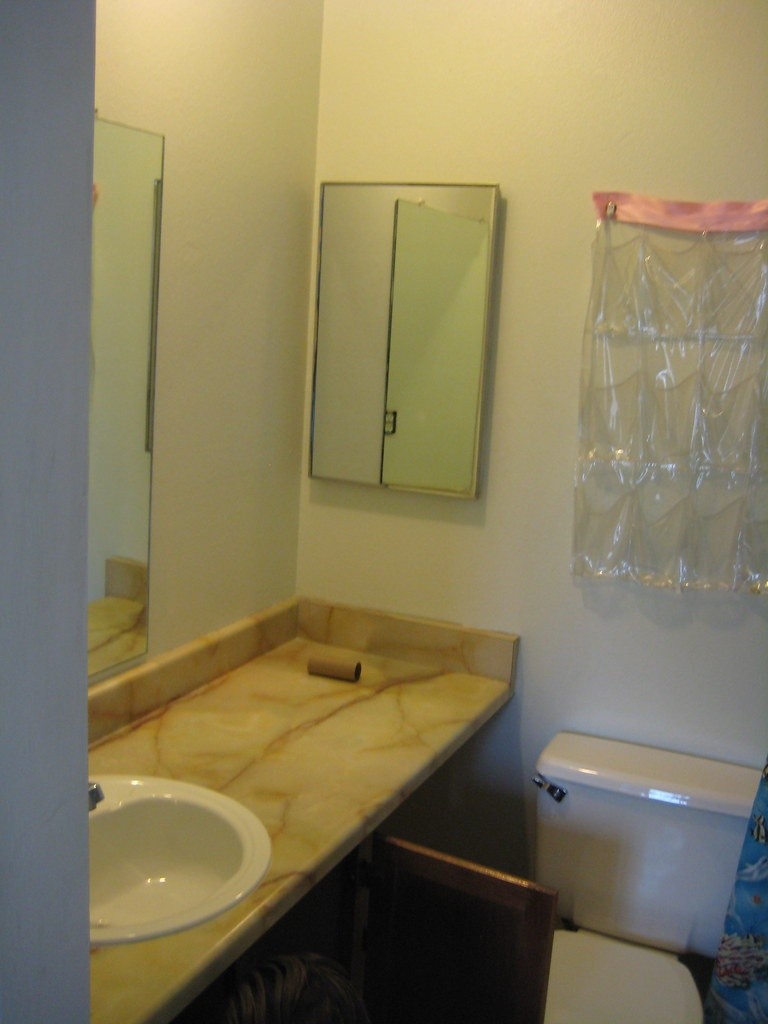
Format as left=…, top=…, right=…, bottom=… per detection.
left=309, top=180, right=497, bottom=495
left=83, top=117, right=165, bottom=681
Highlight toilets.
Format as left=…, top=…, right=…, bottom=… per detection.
left=532, top=731, right=763, bottom=1024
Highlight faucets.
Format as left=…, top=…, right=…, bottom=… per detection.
left=87, top=782, right=105, bottom=811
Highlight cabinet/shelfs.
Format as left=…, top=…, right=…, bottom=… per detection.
left=175, top=830, right=560, bottom=1023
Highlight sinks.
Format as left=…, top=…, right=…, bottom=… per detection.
left=89, top=773, right=272, bottom=945
